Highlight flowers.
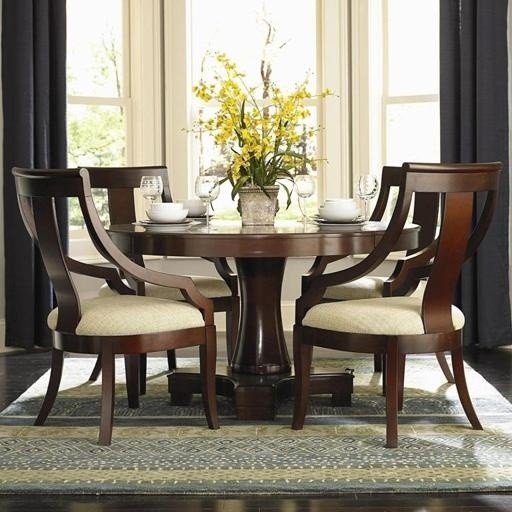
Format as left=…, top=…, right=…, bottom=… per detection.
left=181, top=50, right=339, bottom=211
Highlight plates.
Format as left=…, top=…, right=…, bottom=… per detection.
left=186, top=215, right=214, bottom=220
left=312, top=223, right=367, bottom=230
left=131, top=221, right=202, bottom=232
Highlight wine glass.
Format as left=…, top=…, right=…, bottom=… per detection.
left=139, top=175, right=164, bottom=203
left=195, top=175, right=220, bottom=230
left=355, top=175, right=378, bottom=220
left=295, top=174, right=315, bottom=222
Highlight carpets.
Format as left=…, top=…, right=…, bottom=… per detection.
left=0, top=353, right=511, bottom=497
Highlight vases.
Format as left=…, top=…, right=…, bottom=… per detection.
left=238, top=184, right=280, bottom=226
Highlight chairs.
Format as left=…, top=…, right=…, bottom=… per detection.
left=78, top=165, right=241, bottom=395
left=12, top=167, right=220, bottom=446
left=301, top=166, right=455, bottom=397
left=290, top=161, right=504, bottom=449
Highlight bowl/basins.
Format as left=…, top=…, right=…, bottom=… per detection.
left=324, top=202, right=356, bottom=208
left=318, top=208, right=361, bottom=223
left=176, top=200, right=202, bottom=205
left=183, top=206, right=206, bottom=217
left=151, top=203, right=184, bottom=211
left=326, top=199, right=353, bottom=202
left=144, top=210, right=190, bottom=222
